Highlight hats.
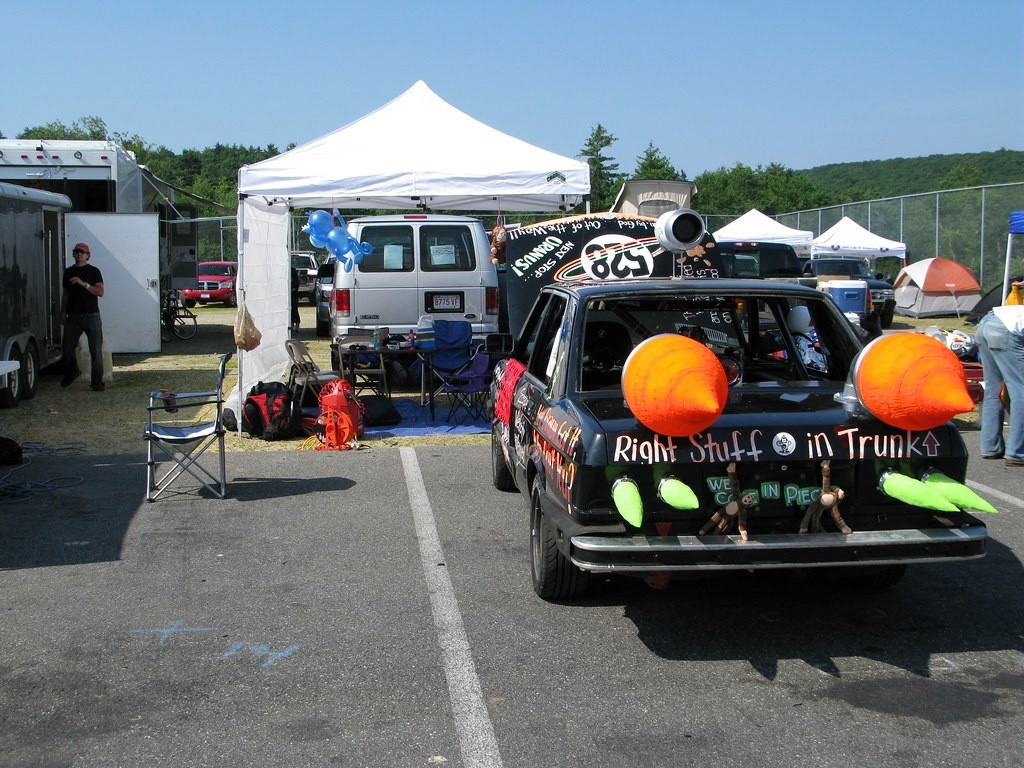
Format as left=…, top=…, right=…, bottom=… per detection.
left=73, top=243, right=88, bottom=253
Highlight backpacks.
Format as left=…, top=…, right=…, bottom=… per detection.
left=343, top=344, right=379, bottom=368
left=242, top=393, right=294, bottom=441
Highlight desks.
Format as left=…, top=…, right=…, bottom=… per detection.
left=338, top=341, right=486, bottom=394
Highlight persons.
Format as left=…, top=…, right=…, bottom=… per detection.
left=291, top=268, right=300, bottom=332
left=975, top=305, right=1024, bottom=467
left=60, top=243, right=105, bottom=391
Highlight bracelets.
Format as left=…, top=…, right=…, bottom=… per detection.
left=83, top=282, right=90, bottom=289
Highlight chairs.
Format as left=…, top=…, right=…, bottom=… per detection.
left=581, top=322, right=636, bottom=392
left=285, top=338, right=340, bottom=425
left=418, top=320, right=484, bottom=408
left=338, top=335, right=390, bottom=403
left=440, top=353, right=491, bottom=425
left=143, top=352, right=233, bottom=501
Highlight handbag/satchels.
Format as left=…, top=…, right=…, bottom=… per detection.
left=418, top=314, right=433, bottom=327
left=241, top=381, right=301, bottom=431
left=234, top=286, right=262, bottom=352
left=358, top=394, right=400, bottom=427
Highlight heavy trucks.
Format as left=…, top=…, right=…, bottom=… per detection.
left=0, top=139, right=163, bottom=408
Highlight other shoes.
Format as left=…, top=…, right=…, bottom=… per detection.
left=61, top=369, right=81, bottom=388
left=1004, top=459, right=1024, bottom=467
left=91, top=381, right=104, bottom=391
left=984, top=456, right=1003, bottom=459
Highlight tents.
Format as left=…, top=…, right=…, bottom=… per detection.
left=1001, top=211, right=1024, bottom=306
left=609, top=178, right=698, bottom=217
left=813, top=216, right=906, bottom=267
left=236, top=79, right=592, bottom=440
left=711, top=208, right=814, bottom=258
left=893, top=258, right=982, bottom=319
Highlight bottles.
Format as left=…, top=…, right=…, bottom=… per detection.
left=409, top=330, right=414, bottom=350
left=1006, top=286, right=1023, bottom=305
left=373, top=327, right=380, bottom=350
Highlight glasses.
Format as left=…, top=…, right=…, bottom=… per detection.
left=74, top=249, right=85, bottom=254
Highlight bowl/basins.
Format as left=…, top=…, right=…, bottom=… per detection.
left=415, top=327, right=436, bottom=349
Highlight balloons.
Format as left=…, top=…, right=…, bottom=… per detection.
left=301, top=208, right=373, bottom=273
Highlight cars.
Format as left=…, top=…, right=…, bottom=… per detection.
left=290, top=250, right=320, bottom=306
left=480, top=268, right=989, bottom=603
left=314, top=252, right=338, bottom=339
left=179, top=261, right=240, bottom=309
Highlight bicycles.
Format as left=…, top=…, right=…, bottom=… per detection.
left=159, top=289, right=198, bottom=343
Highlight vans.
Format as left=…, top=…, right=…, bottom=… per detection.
left=315, top=213, right=508, bottom=383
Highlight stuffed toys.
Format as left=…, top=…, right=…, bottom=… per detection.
left=489, top=225, right=507, bottom=265
